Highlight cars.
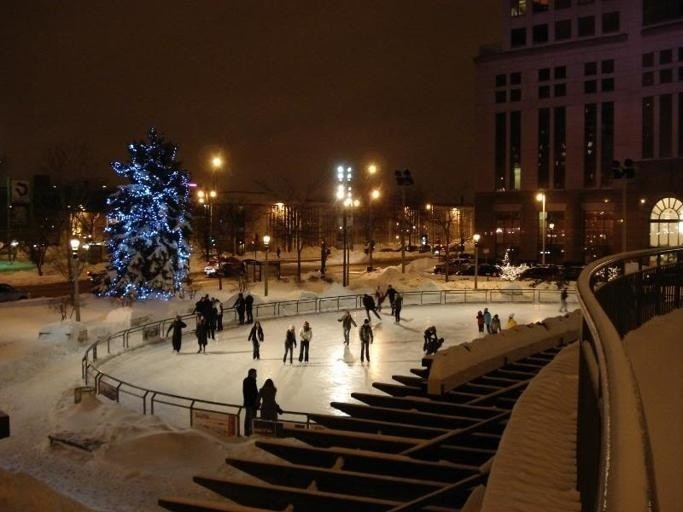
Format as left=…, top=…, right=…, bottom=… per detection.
left=242, top=258, right=261, bottom=272
left=518, top=264, right=597, bottom=281
left=202, top=256, right=244, bottom=279
left=380, top=243, right=502, bottom=279
left=0, top=282, right=32, bottom=303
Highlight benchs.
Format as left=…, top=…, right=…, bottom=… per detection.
left=47, top=431, right=103, bottom=455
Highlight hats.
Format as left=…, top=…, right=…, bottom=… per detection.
left=289, top=325, right=295, bottom=332
left=303, top=321, right=309, bottom=327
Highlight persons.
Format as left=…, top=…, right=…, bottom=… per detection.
left=338, top=311, right=357, bottom=345
left=298, top=321, right=312, bottom=363
left=283, top=326, right=296, bottom=363
left=359, top=319, right=373, bottom=362
left=363, top=284, right=403, bottom=322
left=476, top=308, right=517, bottom=334
left=559, top=288, right=568, bottom=312
left=256, top=379, right=282, bottom=421
left=426, top=338, right=444, bottom=355
left=166, top=293, right=263, bottom=359
left=243, top=369, right=261, bottom=436
left=423, top=327, right=437, bottom=350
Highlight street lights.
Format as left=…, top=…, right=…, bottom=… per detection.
left=212, top=155, right=221, bottom=263
left=472, top=232, right=478, bottom=290
left=395, top=167, right=413, bottom=273
left=261, top=234, right=271, bottom=297
left=364, top=161, right=380, bottom=273
left=68, top=238, right=82, bottom=324
left=333, top=163, right=359, bottom=288
left=536, top=189, right=547, bottom=266
left=196, top=187, right=216, bottom=263
